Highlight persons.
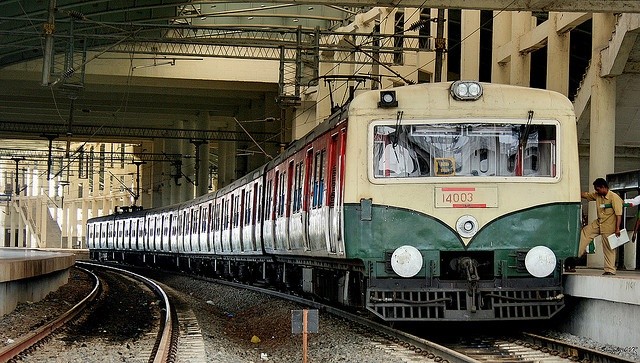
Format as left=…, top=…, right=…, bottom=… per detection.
left=576, top=177, right=621, bottom=278
left=622, top=182, right=640, bottom=209
left=631, top=210, right=638, bottom=274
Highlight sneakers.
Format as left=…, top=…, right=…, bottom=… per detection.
left=602, top=272, right=616, bottom=276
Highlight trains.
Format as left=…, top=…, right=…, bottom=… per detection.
left=85, top=80, right=582, bottom=321
